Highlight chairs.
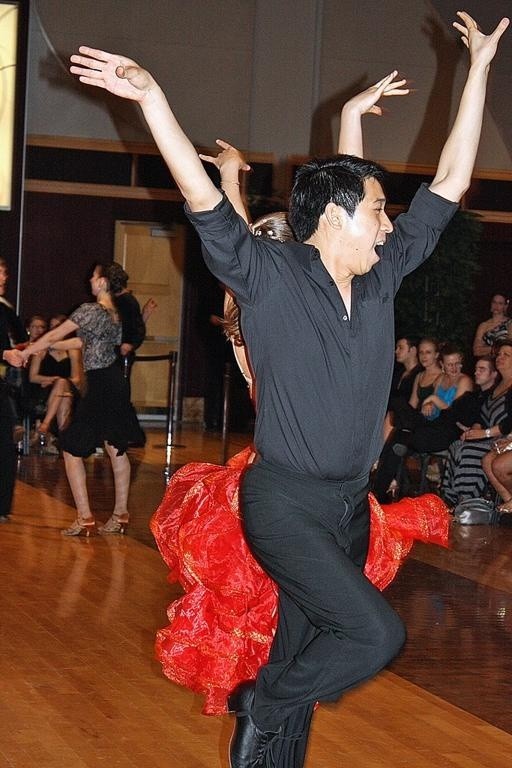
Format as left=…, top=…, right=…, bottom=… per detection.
left=120, top=346, right=183, bottom=480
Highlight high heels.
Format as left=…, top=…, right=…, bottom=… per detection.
left=60, top=512, right=130, bottom=538
left=386, top=486, right=398, bottom=495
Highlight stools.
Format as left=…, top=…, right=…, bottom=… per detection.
left=392, top=446, right=448, bottom=495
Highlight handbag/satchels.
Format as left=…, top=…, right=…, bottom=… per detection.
left=454, top=497, right=501, bottom=525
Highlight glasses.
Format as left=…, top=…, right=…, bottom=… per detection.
left=446, top=361, right=465, bottom=369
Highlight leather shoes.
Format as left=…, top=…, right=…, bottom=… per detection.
left=226, top=680, right=284, bottom=768
